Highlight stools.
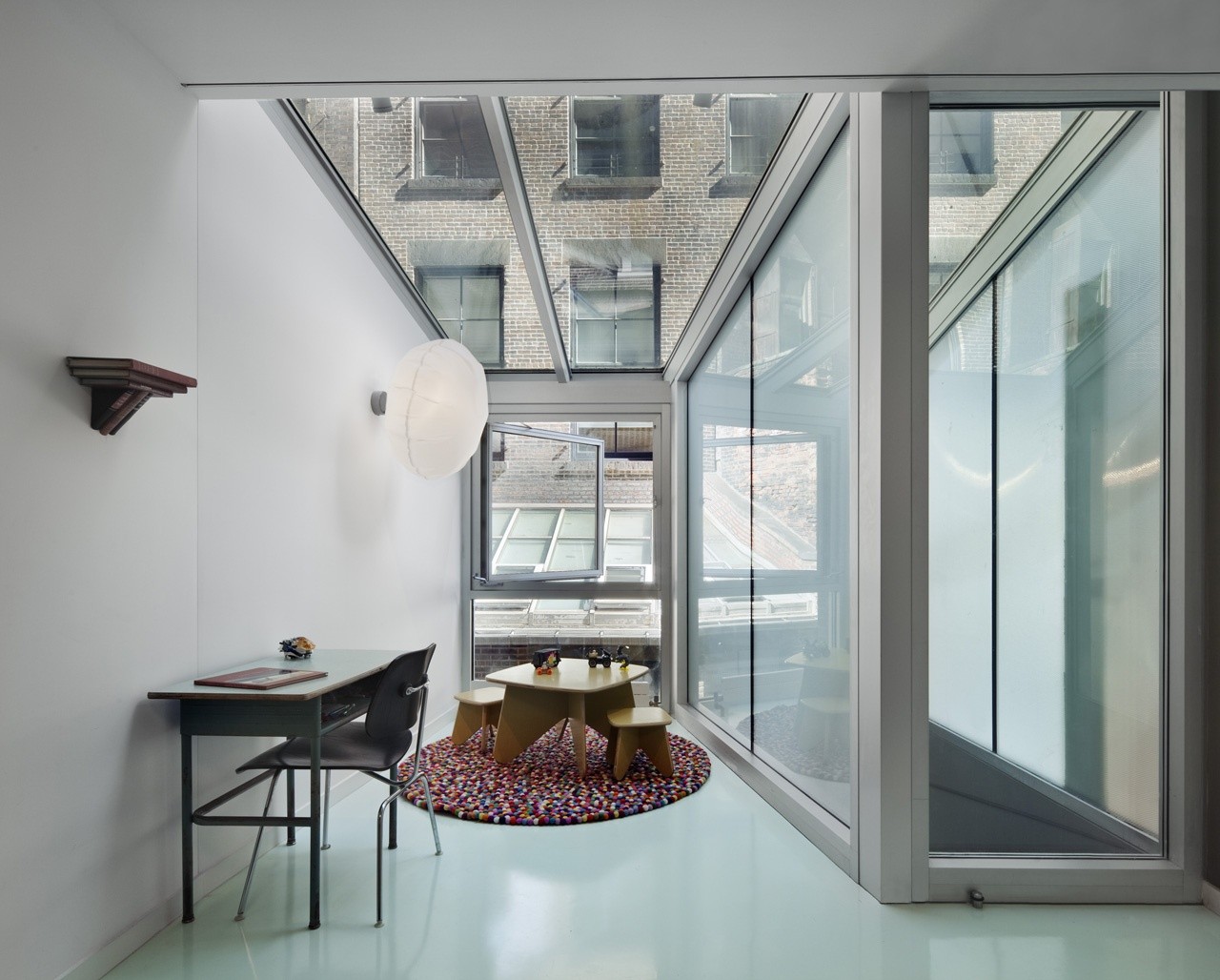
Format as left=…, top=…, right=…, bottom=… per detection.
left=452, top=686, right=505, bottom=756
left=606, top=706, right=674, bottom=780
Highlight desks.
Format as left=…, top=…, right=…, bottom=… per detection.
left=484, top=658, right=651, bottom=780
left=146, top=647, right=422, bottom=931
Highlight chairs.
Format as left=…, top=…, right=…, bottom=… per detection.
left=234, top=643, right=444, bottom=929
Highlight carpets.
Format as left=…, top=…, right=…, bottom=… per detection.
left=738, top=704, right=851, bottom=786
left=395, top=719, right=712, bottom=828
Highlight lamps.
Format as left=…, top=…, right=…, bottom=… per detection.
left=376, top=334, right=491, bottom=480
